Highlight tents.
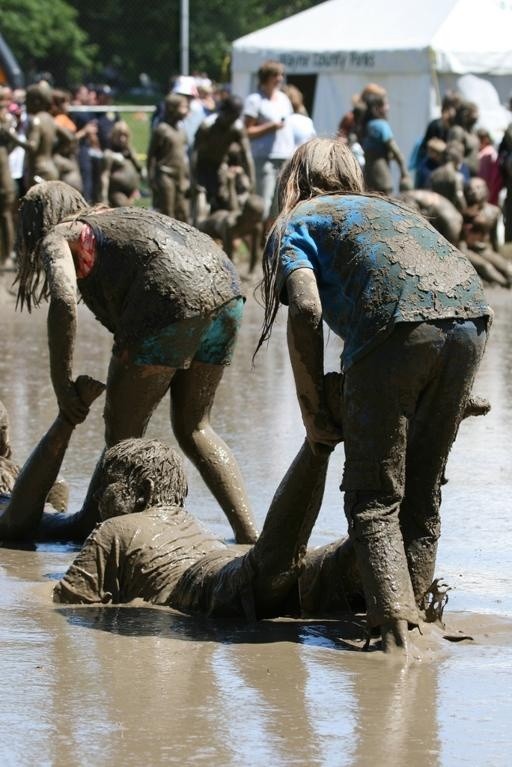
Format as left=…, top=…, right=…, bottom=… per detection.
left=226, top=0, right=512, bottom=197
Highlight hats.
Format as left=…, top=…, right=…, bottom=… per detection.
left=174, top=76, right=201, bottom=98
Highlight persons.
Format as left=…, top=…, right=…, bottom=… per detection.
left=48, top=369, right=491, bottom=639
left=0, top=372, right=108, bottom=551
left=249, top=131, right=499, bottom=663
left=10, top=175, right=263, bottom=548
